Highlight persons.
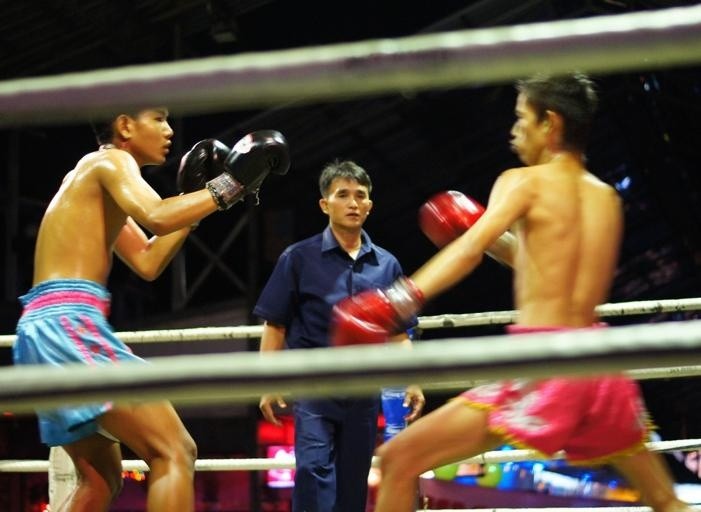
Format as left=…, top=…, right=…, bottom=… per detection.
left=331, top=63, right=698, bottom=512
left=12, top=104, right=296, bottom=511
left=251, top=159, right=426, bottom=512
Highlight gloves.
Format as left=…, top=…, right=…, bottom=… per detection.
left=177, top=136, right=233, bottom=231
left=416, top=187, right=488, bottom=252
left=329, top=274, right=427, bottom=347
left=205, top=128, right=293, bottom=213
